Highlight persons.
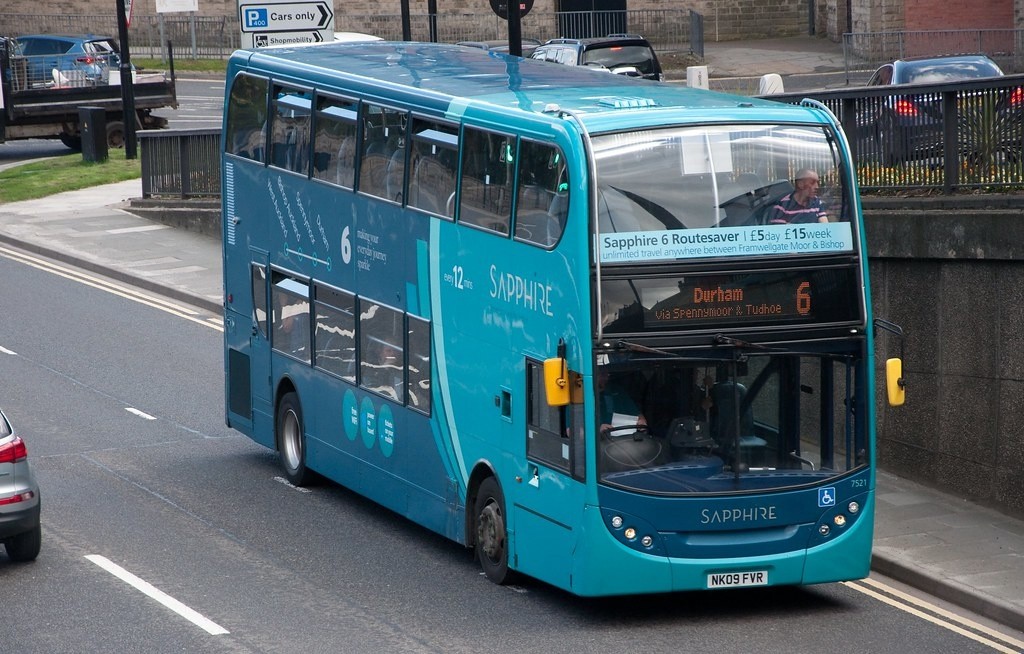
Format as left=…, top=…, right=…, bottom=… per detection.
left=769, top=169, right=829, bottom=225
left=377, top=336, right=397, bottom=366
left=276, top=291, right=305, bottom=333
left=566, top=372, right=647, bottom=438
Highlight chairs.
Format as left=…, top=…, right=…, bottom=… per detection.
left=709, top=381, right=767, bottom=466
left=271, top=305, right=401, bottom=396
left=229, top=112, right=794, bottom=247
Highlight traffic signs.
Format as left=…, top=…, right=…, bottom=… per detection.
left=238, top=1, right=336, bottom=33
left=240, top=33, right=336, bottom=49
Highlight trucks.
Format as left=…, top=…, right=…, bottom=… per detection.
left=0, top=37, right=179, bottom=153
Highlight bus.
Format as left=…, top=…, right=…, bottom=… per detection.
left=220, top=40, right=907, bottom=596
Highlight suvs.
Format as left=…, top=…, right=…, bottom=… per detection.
left=528, top=32, right=663, bottom=86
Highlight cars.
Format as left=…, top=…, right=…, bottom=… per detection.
left=0, top=407, right=43, bottom=563
left=456, top=40, right=542, bottom=59
left=8, top=35, right=136, bottom=87
left=854, top=56, right=1022, bottom=165
